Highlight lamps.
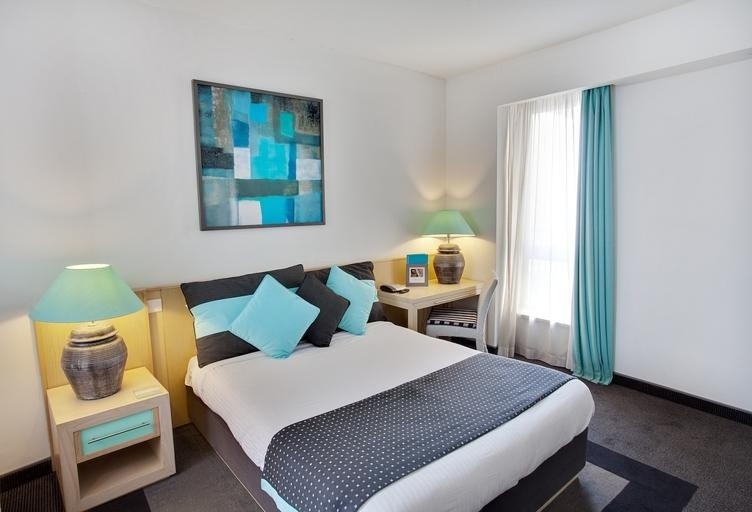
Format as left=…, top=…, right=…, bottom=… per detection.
left=27, top=264, right=145, bottom=401
left=421, top=210, right=475, bottom=285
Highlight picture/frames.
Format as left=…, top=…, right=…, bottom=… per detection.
left=405, top=264, right=429, bottom=288
left=190, top=77, right=326, bottom=232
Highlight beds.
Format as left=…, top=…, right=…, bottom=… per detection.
left=160, top=263, right=595, bottom=511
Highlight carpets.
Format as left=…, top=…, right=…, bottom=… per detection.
left=479, top=438, right=699, bottom=511
left=0, top=461, right=152, bottom=511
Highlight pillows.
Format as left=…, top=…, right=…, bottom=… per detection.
left=230, top=273, right=320, bottom=360
left=180, top=265, right=307, bottom=368
left=303, top=261, right=390, bottom=339
left=294, top=273, right=351, bottom=346
left=324, top=266, right=378, bottom=337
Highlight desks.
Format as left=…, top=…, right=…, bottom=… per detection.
left=379, top=280, right=478, bottom=335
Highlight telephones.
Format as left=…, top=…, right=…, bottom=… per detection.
left=379, top=284, right=409, bottom=294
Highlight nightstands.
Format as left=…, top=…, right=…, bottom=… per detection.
left=44, top=364, right=176, bottom=512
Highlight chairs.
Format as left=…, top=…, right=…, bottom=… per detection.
left=423, top=278, right=500, bottom=353
